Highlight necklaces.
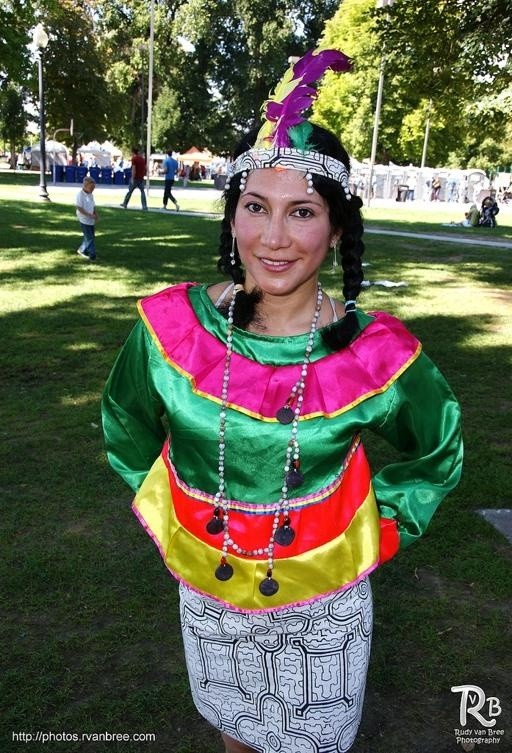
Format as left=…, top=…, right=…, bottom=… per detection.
left=205, top=280, right=323, bottom=596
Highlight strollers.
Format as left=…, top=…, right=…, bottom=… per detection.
left=478, top=196, right=502, bottom=229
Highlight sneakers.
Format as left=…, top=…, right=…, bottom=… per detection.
left=76, top=249, right=97, bottom=263
left=120, top=204, right=180, bottom=212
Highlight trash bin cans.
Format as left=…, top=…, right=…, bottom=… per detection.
left=50, top=165, right=132, bottom=185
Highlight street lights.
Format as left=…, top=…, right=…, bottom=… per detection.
left=137, top=42, right=149, bottom=157
left=30, top=20, right=50, bottom=200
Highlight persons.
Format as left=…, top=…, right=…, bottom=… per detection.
left=161, top=151, right=180, bottom=211
left=466, top=205, right=479, bottom=227
left=120, top=146, right=147, bottom=210
left=100, top=121, right=464, bottom=753
left=75, top=177, right=98, bottom=262
